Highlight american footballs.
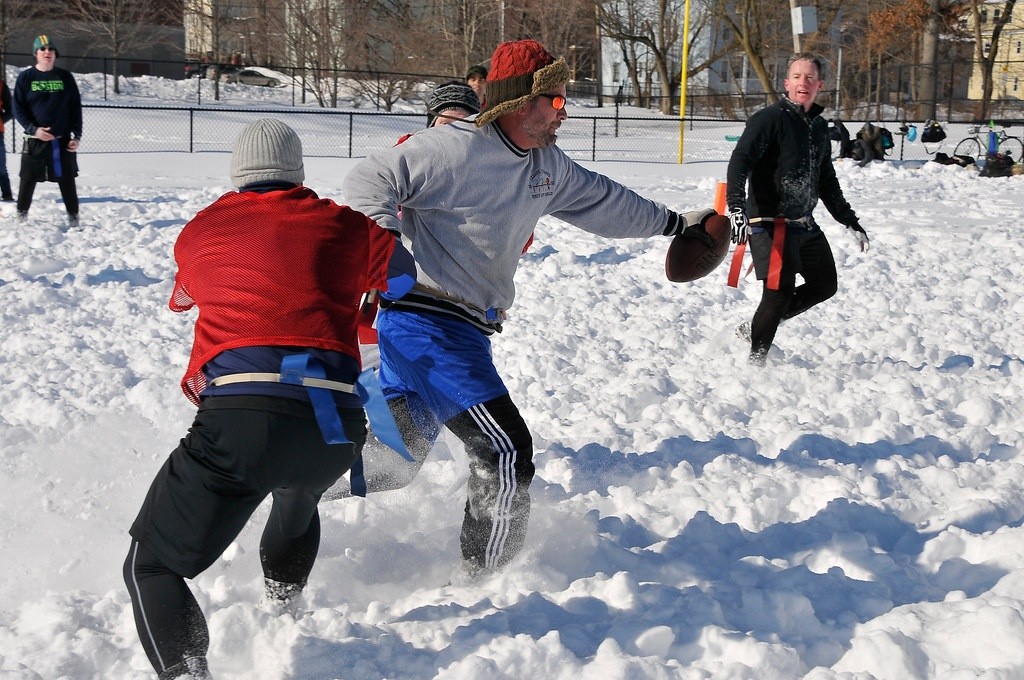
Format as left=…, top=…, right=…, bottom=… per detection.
left=665, top=213, right=732, bottom=283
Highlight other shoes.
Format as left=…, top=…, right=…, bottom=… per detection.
left=735, top=321, right=769, bottom=367
left=15, top=208, right=27, bottom=220
left=2, top=194, right=14, bottom=201
left=69, top=213, right=82, bottom=232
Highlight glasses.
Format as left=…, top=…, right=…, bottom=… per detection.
left=541, top=94, right=567, bottom=111
left=41, top=47, right=55, bottom=51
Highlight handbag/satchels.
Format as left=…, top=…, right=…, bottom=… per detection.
left=920, top=122, right=945, bottom=153
left=981, top=154, right=1015, bottom=177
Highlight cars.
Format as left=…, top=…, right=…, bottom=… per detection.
left=226, top=70, right=280, bottom=87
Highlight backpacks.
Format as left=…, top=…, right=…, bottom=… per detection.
left=880, top=127, right=894, bottom=149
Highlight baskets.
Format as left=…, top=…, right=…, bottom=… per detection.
left=967, top=126, right=980, bottom=134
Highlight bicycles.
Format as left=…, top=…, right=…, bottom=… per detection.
left=954, top=114, right=1024, bottom=163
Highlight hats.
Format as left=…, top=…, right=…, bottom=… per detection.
left=32, top=34, right=60, bottom=59
left=427, top=80, right=481, bottom=131
left=466, top=66, right=487, bottom=82
left=474, top=39, right=572, bottom=128
left=230, top=118, right=305, bottom=189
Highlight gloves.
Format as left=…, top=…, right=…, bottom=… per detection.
left=680, top=208, right=718, bottom=248
left=848, top=221, right=870, bottom=254
left=730, top=206, right=753, bottom=245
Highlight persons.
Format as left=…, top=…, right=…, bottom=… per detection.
left=726, top=51, right=869, bottom=367
left=0, top=80, right=16, bottom=202
left=319, top=40, right=718, bottom=585
left=12, top=35, right=83, bottom=233
left=122, top=120, right=418, bottom=680
left=394, top=66, right=534, bottom=256
